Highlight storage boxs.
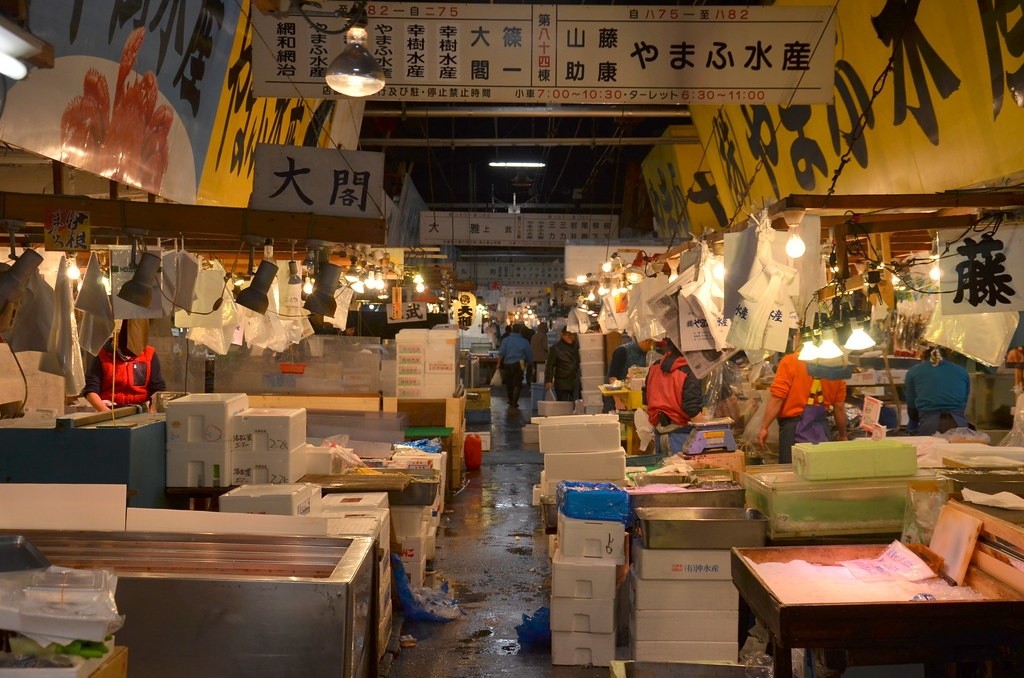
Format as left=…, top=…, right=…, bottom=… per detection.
left=0, top=320, right=1024, bottom=678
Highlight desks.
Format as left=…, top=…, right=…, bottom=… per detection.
left=729, top=545, right=1024, bottom=678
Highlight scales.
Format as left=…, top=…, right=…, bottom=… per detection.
left=681, top=417, right=738, bottom=455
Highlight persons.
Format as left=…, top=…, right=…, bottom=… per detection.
left=339, top=324, right=355, bottom=337
left=495, top=321, right=579, bottom=406
left=903, top=342, right=970, bottom=435
left=81, top=319, right=166, bottom=414
left=602, top=335, right=706, bottom=452
left=757, top=330, right=848, bottom=463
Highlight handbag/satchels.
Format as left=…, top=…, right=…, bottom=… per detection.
left=490, top=369, right=503, bottom=387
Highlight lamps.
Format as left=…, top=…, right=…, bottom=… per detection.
left=0, top=219, right=43, bottom=302
left=117, top=225, right=162, bottom=309
left=237, top=233, right=280, bottom=315
left=489, top=161, right=545, bottom=168
left=301, top=260, right=342, bottom=318
left=288, top=239, right=301, bottom=284
left=624, top=250, right=646, bottom=284
left=288, top=0, right=385, bottom=96
left=796, top=282, right=876, bottom=362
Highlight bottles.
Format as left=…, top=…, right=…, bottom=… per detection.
left=464, top=433, right=482, bottom=470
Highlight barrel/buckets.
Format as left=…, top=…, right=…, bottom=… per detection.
left=530, top=382, right=545, bottom=410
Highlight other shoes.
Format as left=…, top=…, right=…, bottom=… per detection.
left=507, top=400, right=519, bottom=407
